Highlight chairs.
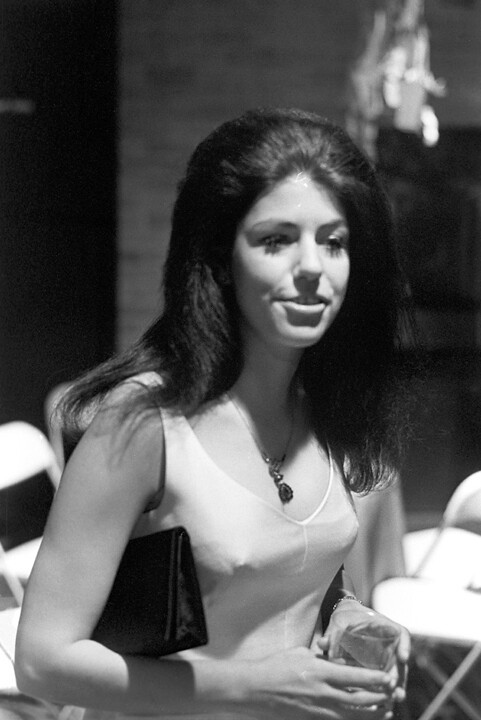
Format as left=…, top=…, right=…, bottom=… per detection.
left=0, top=422, right=63, bottom=608
left=47, top=384, right=97, bottom=470
left=371, top=471, right=481, bottom=720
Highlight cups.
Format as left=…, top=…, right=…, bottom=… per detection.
left=327, top=611, right=401, bottom=711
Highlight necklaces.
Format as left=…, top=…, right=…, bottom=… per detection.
left=227, top=393, right=294, bottom=504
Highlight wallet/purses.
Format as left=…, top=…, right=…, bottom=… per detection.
left=89, top=525, right=209, bottom=658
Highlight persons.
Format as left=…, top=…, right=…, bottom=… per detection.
left=14, top=109, right=412, bottom=720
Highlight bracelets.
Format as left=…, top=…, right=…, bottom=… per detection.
left=332, top=595, right=363, bottom=612
